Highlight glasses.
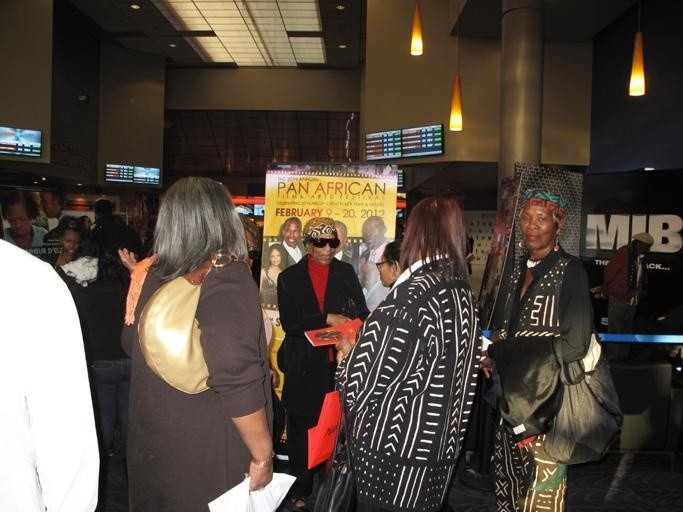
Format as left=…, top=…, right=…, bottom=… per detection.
left=310, top=237, right=340, bottom=248
left=376, top=261, right=388, bottom=272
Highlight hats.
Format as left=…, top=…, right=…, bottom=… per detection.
left=632, top=233, right=653, bottom=245
left=303, top=218, right=337, bottom=247
left=520, top=190, right=570, bottom=219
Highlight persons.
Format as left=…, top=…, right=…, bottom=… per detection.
left=239, top=221, right=261, bottom=286
left=116, top=176, right=273, bottom=512
left=78, top=216, right=92, bottom=230
left=590, top=232, right=652, bottom=365
left=480, top=188, right=624, bottom=512
left=1, top=191, right=47, bottom=248
left=312, top=193, right=479, bottom=512
left=53, top=220, right=99, bottom=294
left=277, top=217, right=370, bottom=508
left=336, top=238, right=406, bottom=366
left=0, top=236, right=100, bottom=511
left=267, top=216, right=302, bottom=267
left=94, top=200, right=144, bottom=359
left=358, top=216, right=391, bottom=264
left=259, top=244, right=288, bottom=309
left=360, top=261, right=390, bottom=312
left=332, top=221, right=352, bottom=263
left=39, top=189, right=63, bottom=219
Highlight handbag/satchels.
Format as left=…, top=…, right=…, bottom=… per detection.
left=307, top=383, right=345, bottom=471
left=310, top=445, right=357, bottom=512
left=207, top=472, right=297, bottom=512
left=137, top=252, right=210, bottom=395
left=542, top=345, right=624, bottom=467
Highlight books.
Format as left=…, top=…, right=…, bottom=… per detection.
left=302, top=317, right=362, bottom=347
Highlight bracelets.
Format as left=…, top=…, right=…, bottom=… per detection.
left=246, top=454, right=276, bottom=467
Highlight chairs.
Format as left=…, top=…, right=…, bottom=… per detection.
left=608, top=362, right=677, bottom=476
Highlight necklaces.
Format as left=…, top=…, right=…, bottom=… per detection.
left=524, top=258, right=542, bottom=270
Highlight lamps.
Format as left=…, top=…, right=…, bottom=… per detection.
left=446, top=1, right=468, bottom=135
left=627, top=1, right=646, bottom=97
left=409, top=1, right=424, bottom=56
